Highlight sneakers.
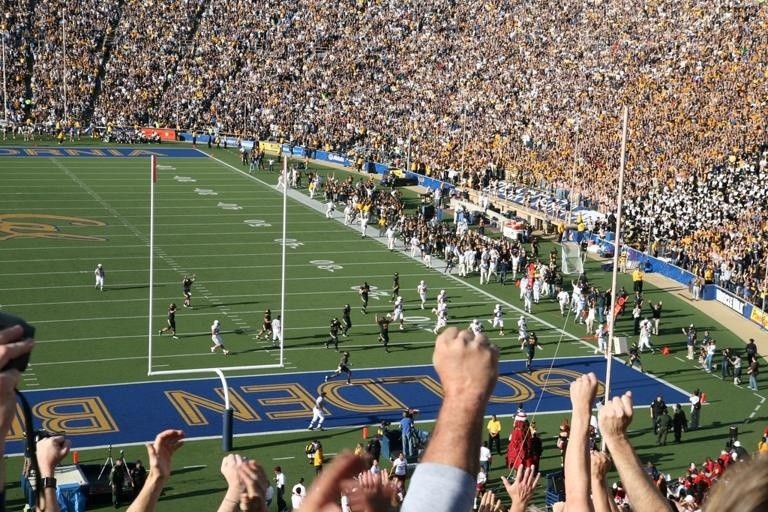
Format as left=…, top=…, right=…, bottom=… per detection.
left=360, top=310, right=366, bottom=315
left=173, top=336, right=179, bottom=339
left=432, top=308, right=436, bottom=315
left=184, top=304, right=186, bottom=308
left=528, top=368, right=531, bottom=375
left=318, top=428, right=322, bottom=430
left=265, top=335, right=269, bottom=339
left=420, top=304, right=424, bottom=309
left=499, top=331, right=504, bottom=336
left=433, top=328, right=438, bottom=335
left=159, top=329, right=161, bottom=336
left=389, top=300, right=395, bottom=303
left=386, top=312, right=391, bottom=318
left=95, top=286, right=98, bottom=289
left=384, top=350, right=390, bottom=353
left=256, top=335, right=260, bottom=339
left=210, top=347, right=214, bottom=352
left=309, top=428, right=313, bottom=430
left=325, top=342, right=328, bottom=349
left=325, top=375, right=328, bottom=382
left=378, top=337, right=382, bottom=342
left=101, top=287, right=103, bottom=291
left=342, top=333, right=348, bottom=338
left=346, top=381, right=353, bottom=386
left=399, top=324, right=404, bottom=330
left=488, top=319, right=492, bottom=324
left=223, top=350, right=226, bottom=352
left=187, top=304, right=192, bottom=307
left=335, top=347, right=339, bottom=351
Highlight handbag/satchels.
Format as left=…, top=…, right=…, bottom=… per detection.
left=695, top=402, right=701, bottom=409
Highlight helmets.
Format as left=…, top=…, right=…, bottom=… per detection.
left=214, top=320, right=220, bottom=325
left=344, top=304, right=349, bottom=308
left=97, top=264, right=102, bottom=267
left=394, top=272, right=398, bottom=275
left=530, top=332, right=535, bottom=338
left=473, top=320, right=478, bottom=323
left=169, top=303, right=176, bottom=308
left=421, top=280, right=425, bottom=284
left=397, top=296, right=403, bottom=302
left=265, top=308, right=270, bottom=314
left=496, top=303, right=502, bottom=309
left=521, top=316, right=525, bottom=321
left=344, top=351, right=350, bottom=357
left=332, top=316, right=338, bottom=321
left=441, top=290, right=446, bottom=294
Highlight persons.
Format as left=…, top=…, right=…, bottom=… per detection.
left=455, top=1, right=666, bottom=233
left=401, top=408, right=424, bottom=462
left=342, top=303, right=352, bottom=337
left=217, top=454, right=243, bottom=512
left=181, top=275, right=195, bottom=307
left=159, top=303, right=178, bottom=340
left=667, top=0, right=768, bottom=307
left=237, top=0, right=455, bottom=275
left=490, top=304, right=504, bottom=335
left=390, top=272, right=400, bottom=302
left=325, top=352, right=354, bottom=386
left=131, top=460, right=146, bottom=494
left=374, top=316, right=393, bottom=352
left=400, top=326, right=500, bottom=512
left=521, top=332, right=543, bottom=374
left=94, top=264, right=105, bottom=291
left=307, top=392, right=325, bottom=431
left=238, top=438, right=408, bottom=512
left=518, top=316, right=527, bottom=341
left=109, top=459, right=123, bottom=509
left=519, top=233, right=573, bottom=316
left=469, top=318, right=483, bottom=334
left=455, top=233, right=540, bottom=285
left=271, top=315, right=283, bottom=343
left=325, top=318, right=344, bottom=351
left=431, top=289, right=448, bottom=334
left=0, top=324, right=34, bottom=512
left=0, top=2, right=237, bottom=147
left=417, top=280, right=428, bottom=309
left=126, top=428, right=184, bottom=512
left=359, top=280, right=370, bottom=315
left=211, top=319, right=230, bottom=356
left=473, top=373, right=768, bottom=512
left=393, top=296, right=404, bottom=331
left=573, top=233, right=664, bottom=372
left=34, top=435, right=71, bottom=512
left=256, top=310, right=270, bottom=342
left=681, top=323, right=759, bottom=391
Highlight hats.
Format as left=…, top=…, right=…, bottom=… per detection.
left=321, top=393, right=326, bottom=395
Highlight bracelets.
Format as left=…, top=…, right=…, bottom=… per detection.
left=224, top=498, right=238, bottom=505
left=40, top=477, right=58, bottom=489
